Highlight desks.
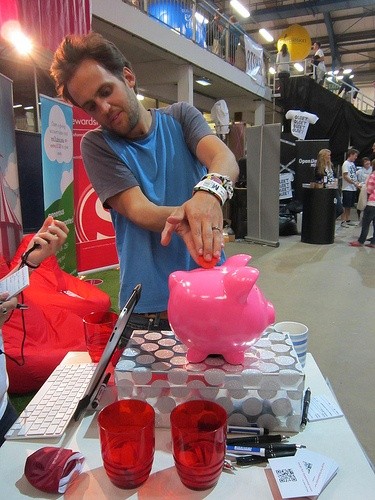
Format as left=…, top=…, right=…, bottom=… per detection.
left=0, top=348, right=373, bottom=500
left=301, top=182, right=342, bottom=246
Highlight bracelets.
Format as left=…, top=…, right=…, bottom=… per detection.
left=192, top=171, right=235, bottom=207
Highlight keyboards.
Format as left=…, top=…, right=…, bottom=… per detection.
left=4, top=363, right=98, bottom=439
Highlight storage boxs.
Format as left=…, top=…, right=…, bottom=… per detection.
left=114, top=332, right=305, bottom=433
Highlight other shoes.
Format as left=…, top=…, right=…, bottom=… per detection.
left=367, top=237, right=373, bottom=242
left=341, top=221, right=349, bottom=229
left=348, top=221, right=358, bottom=226
left=350, top=240, right=363, bottom=247
left=365, top=244, right=375, bottom=248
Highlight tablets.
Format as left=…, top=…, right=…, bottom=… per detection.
left=74, top=282, right=142, bottom=422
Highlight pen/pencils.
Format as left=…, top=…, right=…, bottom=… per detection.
left=223, top=460, right=237, bottom=471
left=0, top=301, right=30, bottom=310
left=301, top=386, right=311, bottom=428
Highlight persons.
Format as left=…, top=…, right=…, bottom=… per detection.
left=310, top=41, right=326, bottom=85
left=275, top=43, right=291, bottom=77
left=353, top=157, right=372, bottom=221
left=370, top=141, right=375, bottom=170
left=0, top=211, right=70, bottom=446
left=207, top=8, right=223, bottom=59
left=223, top=14, right=242, bottom=65
left=337, top=66, right=354, bottom=104
left=340, top=149, right=359, bottom=229
left=50, top=30, right=243, bottom=271
left=349, top=159, right=375, bottom=249
left=314, top=148, right=335, bottom=182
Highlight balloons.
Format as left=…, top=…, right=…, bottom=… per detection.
left=276, top=23, right=312, bottom=61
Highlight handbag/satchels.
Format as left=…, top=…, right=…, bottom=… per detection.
left=352, top=85, right=360, bottom=99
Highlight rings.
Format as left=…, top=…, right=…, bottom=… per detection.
left=212, top=225, right=222, bottom=234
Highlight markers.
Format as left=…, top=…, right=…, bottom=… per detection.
left=225, top=434, right=297, bottom=465
left=92, top=373, right=111, bottom=408
left=226, top=424, right=269, bottom=435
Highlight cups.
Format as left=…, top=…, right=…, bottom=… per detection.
left=83, top=311, right=118, bottom=363
left=275, top=321, right=308, bottom=369
left=98, top=399, right=156, bottom=489
left=171, top=400, right=228, bottom=490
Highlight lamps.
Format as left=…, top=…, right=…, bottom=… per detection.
left=196, top=77, right=214, bottom=87
